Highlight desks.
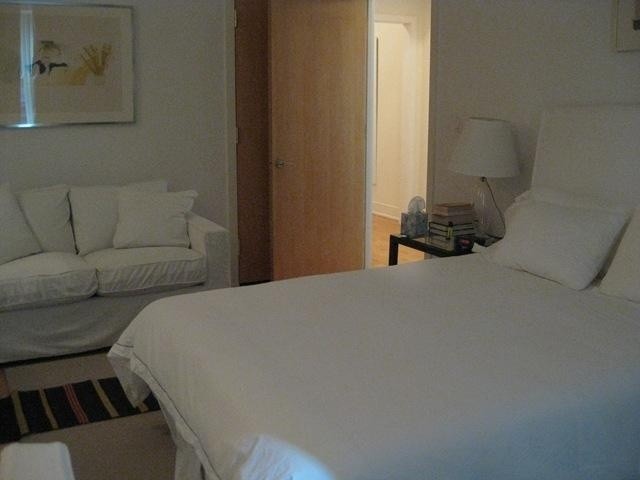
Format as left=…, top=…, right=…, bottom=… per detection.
left=388, top=232, right=502, bottom=267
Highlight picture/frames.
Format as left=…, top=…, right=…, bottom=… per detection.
left=0, top=1, right=135, bottom=130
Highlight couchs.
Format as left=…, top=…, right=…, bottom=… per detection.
left=0, top=183, right=235, bottom=366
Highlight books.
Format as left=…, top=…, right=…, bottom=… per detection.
left=429, top=200, right=479, bottom=249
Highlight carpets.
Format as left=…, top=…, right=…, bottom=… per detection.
left=1, top=375, right=157, bottom=443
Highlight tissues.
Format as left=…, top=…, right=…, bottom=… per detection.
left=401, top=196, right=428, bottom=239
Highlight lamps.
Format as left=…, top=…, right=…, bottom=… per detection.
left=447, top=117, right=519, bottom=237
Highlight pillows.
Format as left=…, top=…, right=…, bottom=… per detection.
left=488, top=182, right=640, bottom=301
left=1, top=179, right=198, bottom=267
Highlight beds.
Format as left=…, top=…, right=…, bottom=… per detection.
left=109, top=103, right=640, bottom=480
